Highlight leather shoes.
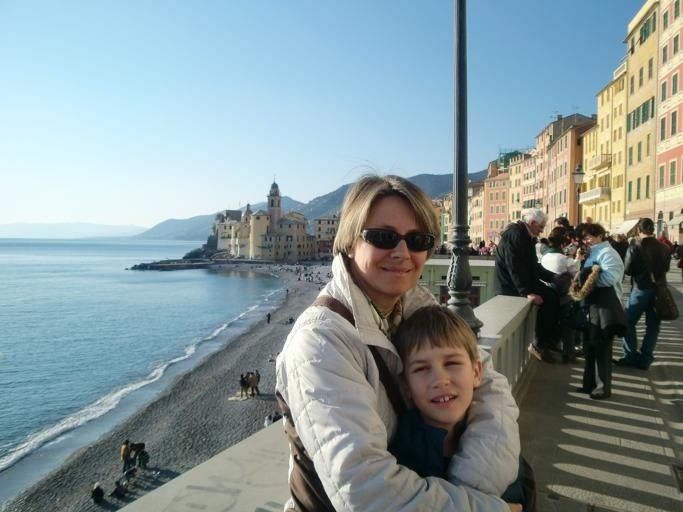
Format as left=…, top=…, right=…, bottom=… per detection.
left=526, top=342, right=561, bottom=363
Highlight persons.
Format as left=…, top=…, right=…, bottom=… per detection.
left=280, top=262, right=333, bottom=290
left=388, top=305, right=536, bottom=512
left=478, top=240, right=498, bottom=256
left=275, top=173, right=524, bottom=512
left=264, top=411, right=282, bottom=428
left=91, top=482, right=104, bottom=503
left=574, top=223, right=624, bottom=398
left=495, top=208, right=683, bottom=368
left=266, top=312, right=271, bottom=323
left=289, top=317, right=294, bottom=324
left=120, top=440, right=149, bottom=482
left=109, top=481, right=128, bottom=499
left=239, top=369, right=260, bottom=398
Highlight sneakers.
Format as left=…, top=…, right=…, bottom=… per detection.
left=591, top=388, right=611, bottom=398
left=577, top=388, right=590, bottom=393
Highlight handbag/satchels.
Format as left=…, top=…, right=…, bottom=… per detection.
left=655, top=285, right=678, bottom=320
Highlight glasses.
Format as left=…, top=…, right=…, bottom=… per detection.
left=359, top=228, right=435, bottom=251
